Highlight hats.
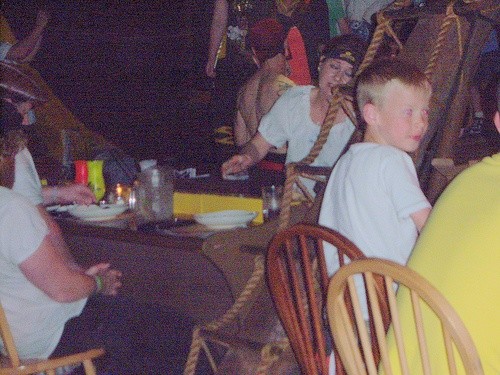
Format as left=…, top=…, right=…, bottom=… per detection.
left=323, top=34, right=368, bottom=65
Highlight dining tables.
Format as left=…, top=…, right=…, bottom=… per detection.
left=45, top=193, right=321, bottom=375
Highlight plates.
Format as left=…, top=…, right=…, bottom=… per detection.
left=67, top=203, right=126, bottom=221
left=194, top=209, right=256, bottom=230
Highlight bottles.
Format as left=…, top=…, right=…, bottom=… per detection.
left=74, top=160, right=106, bottom=202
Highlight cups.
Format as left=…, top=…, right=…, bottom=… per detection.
left=261, top=184, right=285, bottom=223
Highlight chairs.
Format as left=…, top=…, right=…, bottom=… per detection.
left=0, top=303, right=106, bottom=375
left=266, top=222, right=393, bottom=375
left=326, top=257, right=485, bottom=375
left=182, top=218, right=321, bottom=375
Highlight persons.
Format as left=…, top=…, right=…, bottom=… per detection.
left=205, top=0, right=500, bottom=375
left=0, top=8, right=212, bottom=375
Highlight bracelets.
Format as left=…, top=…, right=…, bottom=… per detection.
left=96, top=276, right=102, bottom=294
left=52, top=187, right=57, bottom=202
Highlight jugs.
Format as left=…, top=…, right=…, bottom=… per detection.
left=129, top=165, right=173, bottom=224
